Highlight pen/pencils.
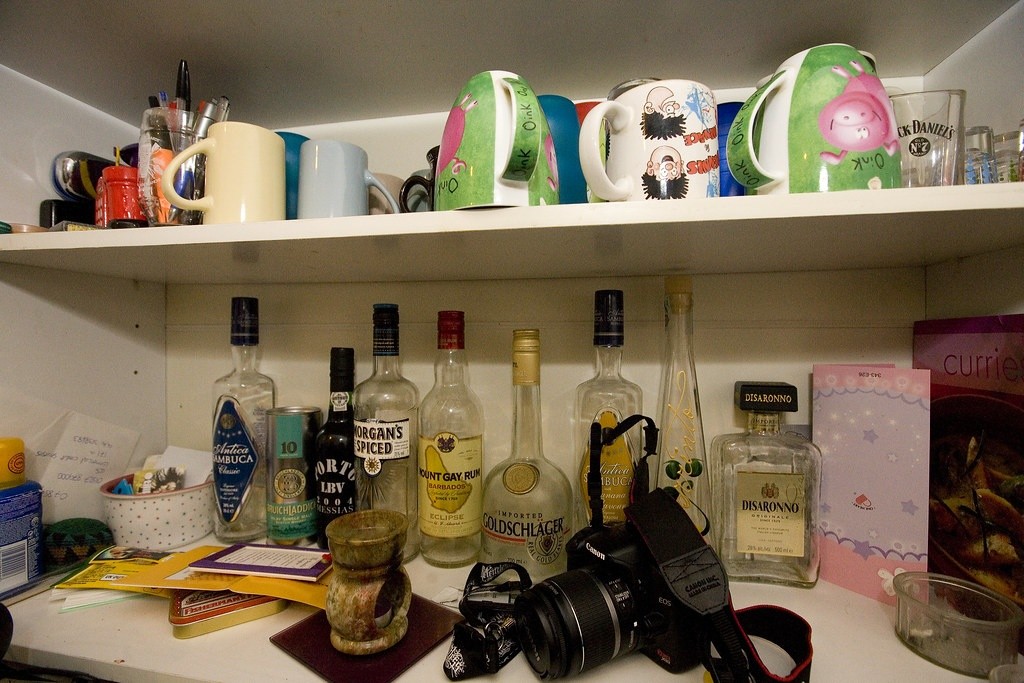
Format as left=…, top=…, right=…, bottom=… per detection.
left=176, top=59, right=190, bottom=152
left=217, top=96, right=232, bottom=121
left=160, top=92, right=169, bottom=108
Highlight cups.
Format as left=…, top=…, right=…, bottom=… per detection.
left=888, top=89, right=966, bottom=188
left=578, top=79, right=720, bottom=200
left=716, top=102, right=757, bottom=197
left=725, top=43, right=900, bottom=195
left=297, top=139, right=399, bottom=219
left=103, top=166, right=147, bottom=222
left=137, top=107, right=207, bottom=227
left=991, top=130, right=1024, bottom=182
left=964, top=126, right=999, bottom=184
left=434, top=70, right=559, bottom=210
left=161, top=121, right=285, bottom=224
left=537, top=95, right=617, bottom=205
left=893, top=572, right=1024, bottom=683
left=400, top=145, right=441, bottom=213
left=274, top=132, right=311, bottom=220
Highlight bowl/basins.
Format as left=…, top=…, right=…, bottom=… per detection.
left=98, top=473, right=214, bottom=549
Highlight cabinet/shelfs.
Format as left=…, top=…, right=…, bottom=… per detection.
left=0, top=1, right=1024, bottom=682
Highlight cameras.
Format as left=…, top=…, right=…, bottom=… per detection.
left=514, top=487, right=730, bottom=683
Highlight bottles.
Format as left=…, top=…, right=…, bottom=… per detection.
left=484, top=329, right=573, bottom=589
left=352, top=303, right=419, bottom=565
left=573, top=290, right=642, bottom=532
left=712, top=381, right=822, bottom=589
left=417, top=310, right=483, bottom=569
left=0, top=436, right=43, bottom=600
left=312, top=347, right=370, bottom=550
left=649, top=275, right=717, bottom=556
left=211, top=296, right=275, bottom=543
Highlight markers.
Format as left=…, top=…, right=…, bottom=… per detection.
left=148, top=96, right=172, bottom=151
left=168, top=103, right=217, bottom=221
